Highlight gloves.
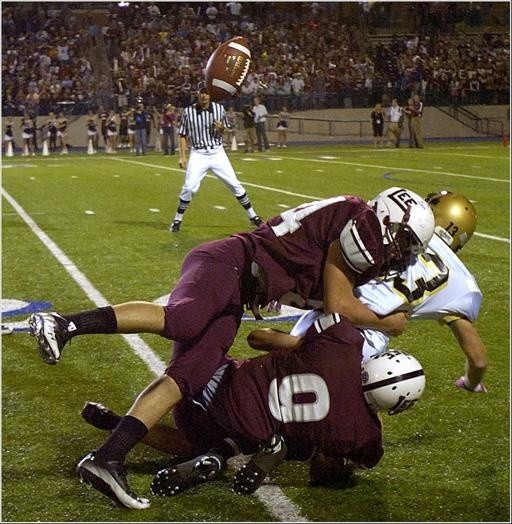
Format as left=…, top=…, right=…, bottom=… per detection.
left=454, top=371, right=488, bottom=394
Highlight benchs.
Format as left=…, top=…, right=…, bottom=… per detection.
left=368, top=33, right=419, bottom=83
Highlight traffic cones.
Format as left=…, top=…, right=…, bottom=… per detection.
left=153, top=136, right=163, bottom=153
left=40, top=140, right=51, bottom=157
left=86, top=138, right=94, bottom=155
left=6, top=141, right=16, bottom=158
left=229, top=135, right=240, bottom=152
left=21, top=143, right=30, bottom=156
left=104, top=138, right=113, bottom=154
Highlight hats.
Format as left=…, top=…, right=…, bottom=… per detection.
left=197, top=81, right=207, bottom=92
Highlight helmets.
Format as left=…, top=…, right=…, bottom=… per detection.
left=359, top=348, right=425, bottom=416
left=366, top=186, right=436, bottom=266
left=426, top=189, right=478, bottom=254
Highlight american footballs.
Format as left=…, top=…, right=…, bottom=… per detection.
left=204, top=35, right=253, bottom=101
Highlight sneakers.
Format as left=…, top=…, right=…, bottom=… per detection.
left=248, top=216, right=263, bottom=228
left=168, top=220, right=181, bottom=233
left=27, top=311, right=70, bottom=366
left=80, top=400, right=121, bottom=432
left=75, top=451, right=152, bottom=510
left=307, top=457, right=359, bottom=490
left=149, top=452, right=220, bottom=497
left=232, top=432, right=280, bottom=498
